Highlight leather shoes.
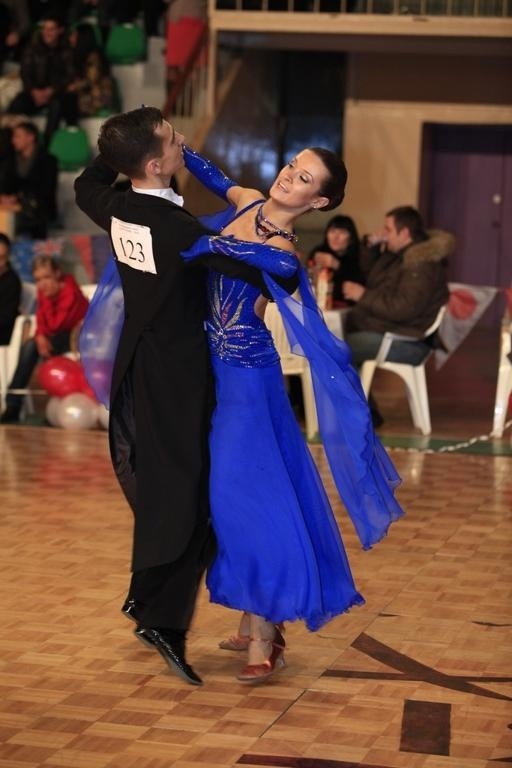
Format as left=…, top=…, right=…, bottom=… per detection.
left=122, top=597, right=205, bottom=685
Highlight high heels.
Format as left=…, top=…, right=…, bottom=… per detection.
left=219, top=632, right=253, bottom=652
left=239, top=628, right=287, bottom=680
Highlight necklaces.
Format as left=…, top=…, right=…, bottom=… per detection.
left=254, top=205, right=297, bottom=244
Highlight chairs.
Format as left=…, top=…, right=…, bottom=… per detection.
left=0, top=280, right=123, bottom=428
left=80, top=77, right=120, bottom=117
left=71, top=19, right=145, bottom=65
left=49, top=127, right=90, bottom=173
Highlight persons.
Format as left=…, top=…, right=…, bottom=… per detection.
left=139, top=103, right=410, bottom=681
left=71, top=102, right=300, bottom=684
left=301, top=204, right=458, bottom=430
left=0, top=1, right=147, bottom=432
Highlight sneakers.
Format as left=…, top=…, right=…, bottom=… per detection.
left=1, top=410, right=20, bottom=423
